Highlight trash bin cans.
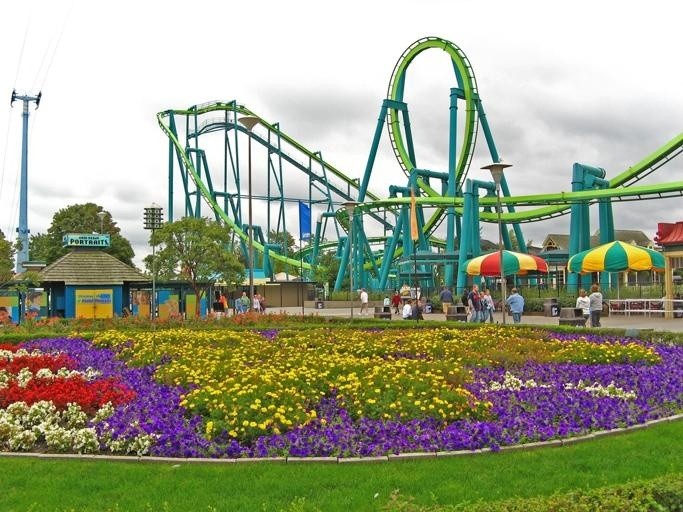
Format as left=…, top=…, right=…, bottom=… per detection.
left=558, top=308, right=586, bottom=326
left=374, top=306, right=392, bottom=320
left=422, top=303, right=432, bottom=313
left=543, top=298, right=559, bottom=317
left=445, top=306, right=468, bottom=322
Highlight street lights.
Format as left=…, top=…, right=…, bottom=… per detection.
left=478, top=162, right=514, bottom=328
left=142, top=202, right=164, bottom=321
left=236, top=114, right=262, bottom=314
left=339, top=199, right=363, bottom=323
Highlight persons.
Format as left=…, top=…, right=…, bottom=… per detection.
left=218, top=294, right=228, bottom=318
left=391, top=291, right=402, bottom=314
left=411, top=300, right=421, bottom=321
left=257, top=293, right=264, bottom=314
left=461, top=284, right=495, bottom=323
left=0, top=307, right=12, bottom=323
left=28, top=291, right=43, bottom=314
left=131, top=289, right=152, bottom=304
left=240, top=291, right=250, bottom=312
left=588, top=284, right=602, bottom=327
left=401, top=299, right=412, bottom=320
left=438, top=286, right=452, bottom=314
left=504, top=287, right=525, bottom=324
left=383, top=296, right=390, bottom=320
left=234, top=297, right=242, bottom=313
left=253, top=295, right=259, bottom=315
left=574, top=287, right=590, bottom=328
left=417, top=296, right=426, bottom=321
left=357, top=287, right=368, bottom=315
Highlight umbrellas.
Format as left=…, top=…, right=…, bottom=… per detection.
left=565, top=240, right=667, bottom=307
left=459, top=249, right=548, bottom=323
